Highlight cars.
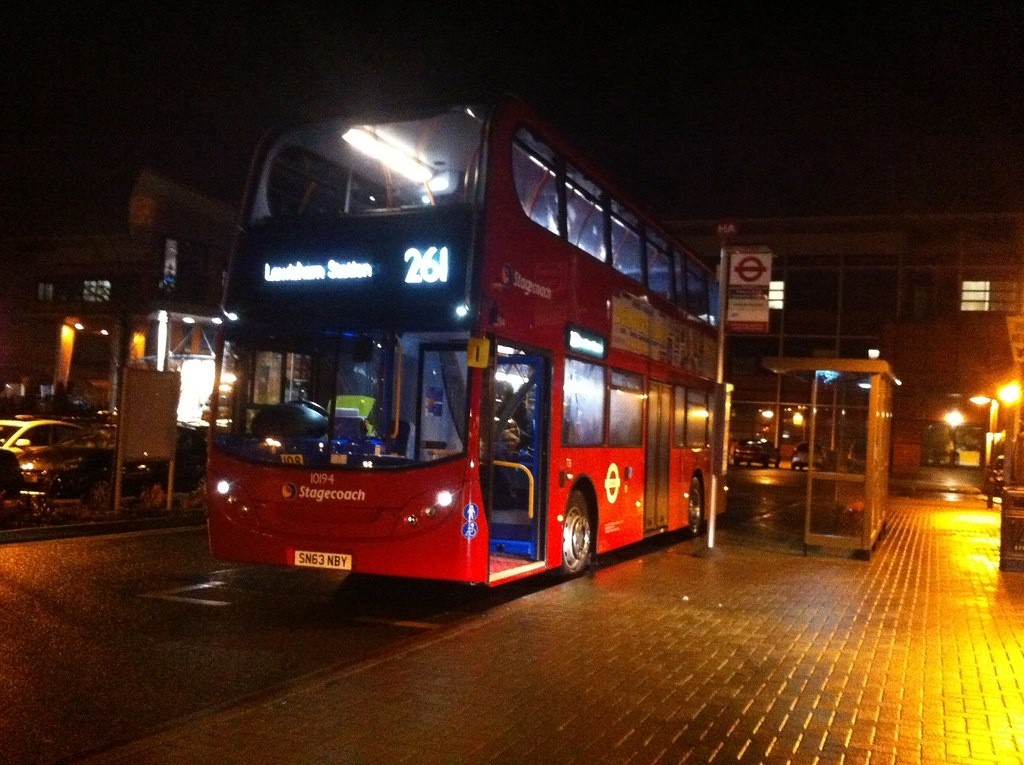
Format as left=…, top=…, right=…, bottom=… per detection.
left=792, top=441, right=831, bottom=472
left=0, top=393, right=207, bottom=507
left=733, top=437, right=781, bottom=468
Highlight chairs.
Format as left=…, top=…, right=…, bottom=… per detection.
left=328, top=395, right=373, bottom=439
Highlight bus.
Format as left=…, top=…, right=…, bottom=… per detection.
left=203, top=81, right=735, bottom=594
